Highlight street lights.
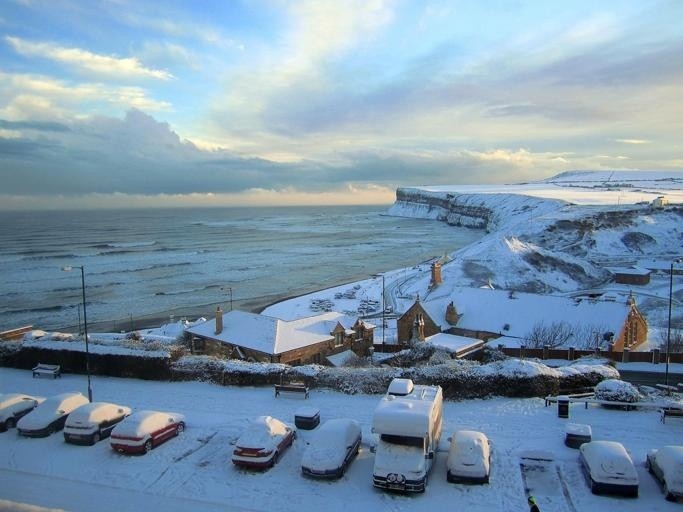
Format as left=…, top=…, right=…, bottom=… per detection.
left=61, top=265, right=92, bottom=403
left=369, top=274, right=384, bottom=354
left=664, top=257, right=682, bottom=386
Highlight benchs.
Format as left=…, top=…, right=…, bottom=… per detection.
left=273, top=373, right=314, bottom=399
left=660, top=407, right=683, bottom=424
left=32, top=362, right=63, bottom=379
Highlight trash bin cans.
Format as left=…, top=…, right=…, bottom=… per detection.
left=558, top=396, right=569, bottom=418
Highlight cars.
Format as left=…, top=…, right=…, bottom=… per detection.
left=644, top=446, right=682, bottom=503
left=301, top=419, right=361, bottom=480
left=109, top=410, right=184, bottom=455
left=578, top=441, right=638, bottom=498
left=446, top=430, right=493, bottom=485
left=63, top=402, right=131, bottom=446
left=16, top=391, right=90, bottom=438
left=0, top=393, right=37, bottom=432
left=231, top=416, right=297, bottom=471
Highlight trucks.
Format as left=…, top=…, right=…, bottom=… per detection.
left=371, top=377, right=443, bottom=494
